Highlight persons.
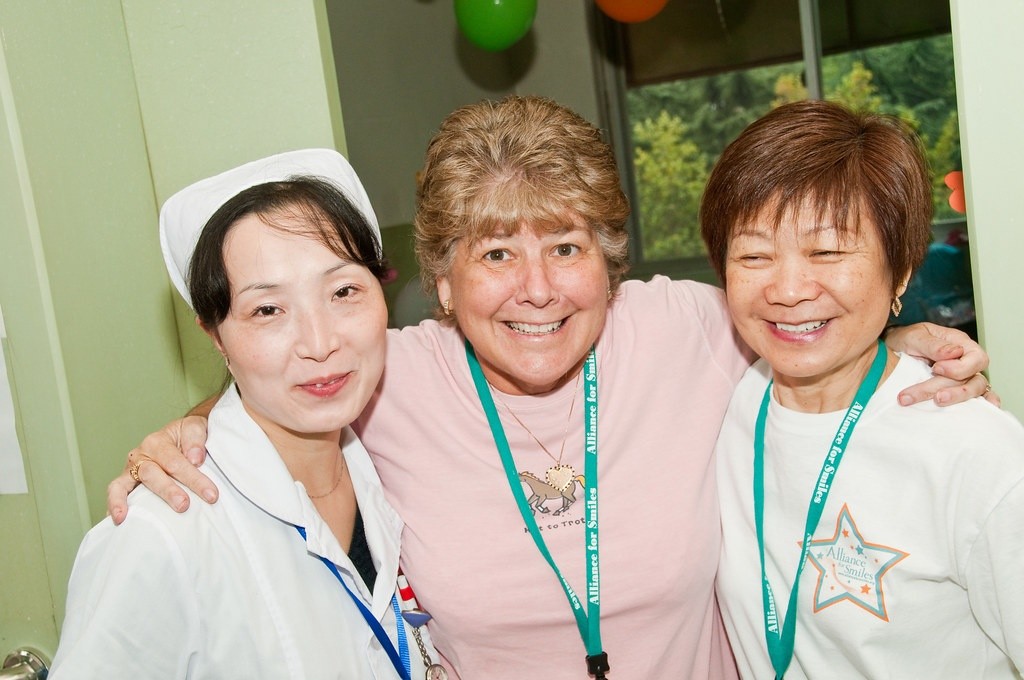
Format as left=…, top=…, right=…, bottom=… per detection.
left=45, top=147, right=441, bottom=680
left=685, top=101, right=1024, bottom=680
left=104, top=95, right=1001, bottom=680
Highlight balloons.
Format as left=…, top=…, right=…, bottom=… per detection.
left=455, top=0, right=538, bottom=53
left=595, top=0, right=668, bottom=23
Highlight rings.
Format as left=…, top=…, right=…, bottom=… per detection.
left=976, top=371, right=992, bottom=396
left=130, top=457, right=151, bottom=482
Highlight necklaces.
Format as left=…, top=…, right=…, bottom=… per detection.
left=309, top=453, right=344, bottom=500
left=486, top=370, right=579, bottom=491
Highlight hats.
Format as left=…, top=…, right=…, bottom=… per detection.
left=158, top=148, right=384, bottom=316
